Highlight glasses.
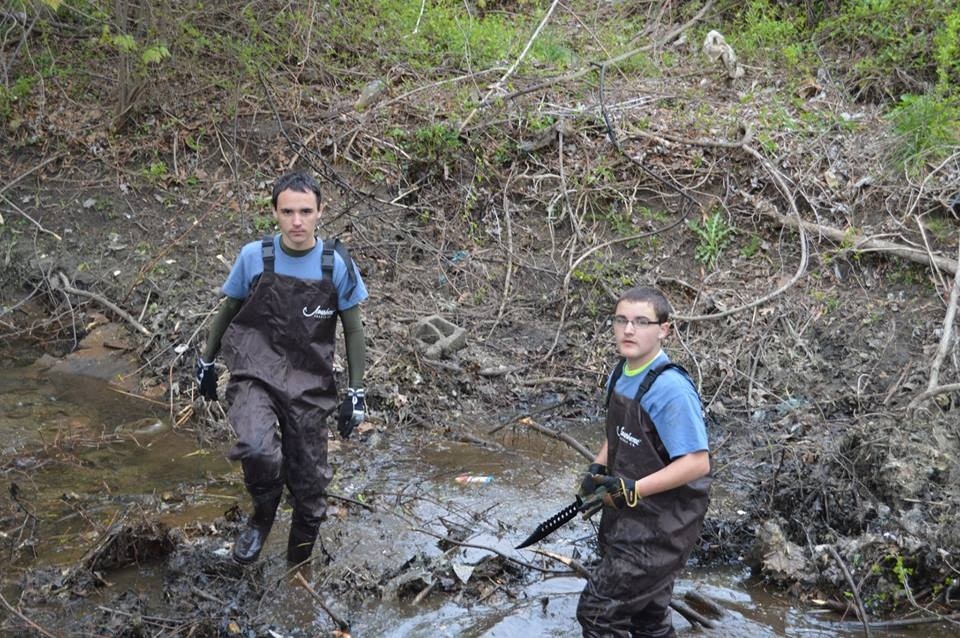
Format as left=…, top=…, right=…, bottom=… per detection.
left=613, top=314, right=664, bottom=330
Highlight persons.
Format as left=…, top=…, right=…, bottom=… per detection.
left=194, top=173, right=369, bottom=566
left=576, top=285, right=712, bottom=638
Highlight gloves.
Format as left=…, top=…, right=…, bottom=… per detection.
left=578, top=471, right=641, bottom=519
left=195, top=358, right=218, bottom=402
left=336, top=388, right=367, bottom=439
left=576, top=462, right=607, bottom=497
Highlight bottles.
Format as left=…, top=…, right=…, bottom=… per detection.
left=455, top=476, right=494, bottom=485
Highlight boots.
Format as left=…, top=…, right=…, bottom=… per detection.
left=287, top=526, right=319, bottom=566
left=233, top=482, right=283, bottom=565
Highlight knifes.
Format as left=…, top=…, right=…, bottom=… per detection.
left=514, top=484, right=607, bottom=549
